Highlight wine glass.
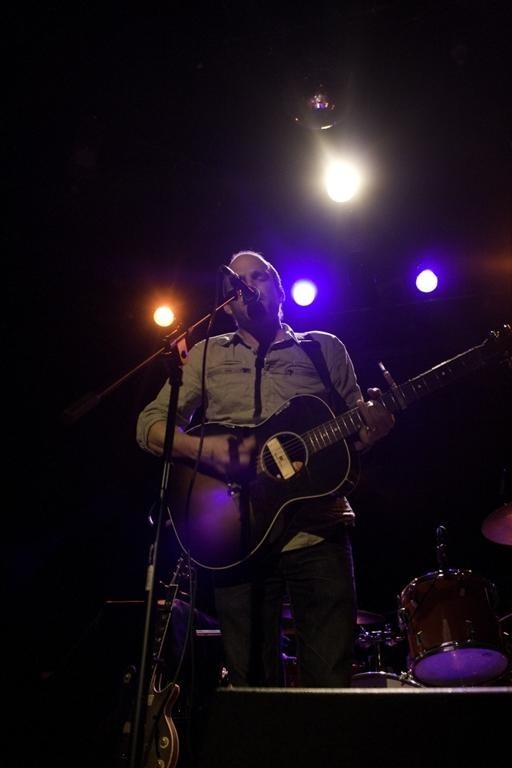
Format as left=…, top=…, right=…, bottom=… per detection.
left=370, top=425, right=378, bottom=434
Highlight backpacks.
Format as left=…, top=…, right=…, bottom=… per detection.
left=283, top=655, right=359, bottom=688
left=352, top=671, right=424, bottom=687
left=397, top=569, right=509, bottom=684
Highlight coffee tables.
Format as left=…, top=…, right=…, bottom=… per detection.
left=281, top=601, right=381, bottom=626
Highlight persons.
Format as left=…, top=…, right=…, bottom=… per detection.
left=136, top=248, right=399, bottom=692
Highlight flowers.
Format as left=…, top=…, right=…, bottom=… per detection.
left=223, top=263, right=260, bottom=306
